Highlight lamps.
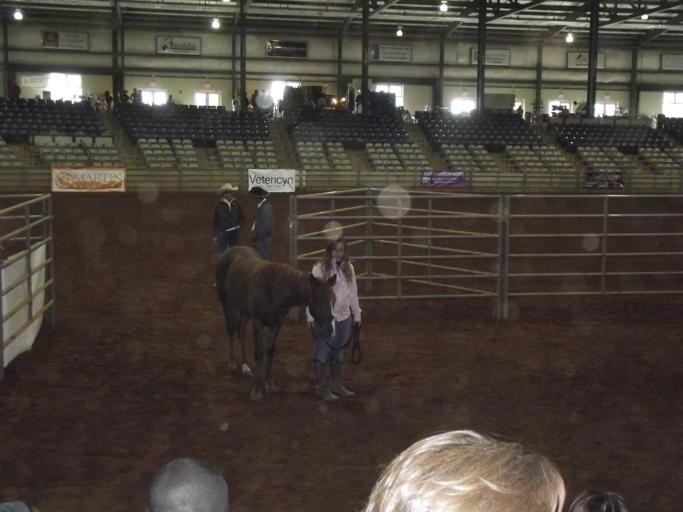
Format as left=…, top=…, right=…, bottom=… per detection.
left=14, top=8, right=23, bottom=21
left=396, top=24, right=404, bottom=37
left=212, top=16, right=220, bottom=29
left=567, top=33, right=573, bottom=42
left=440, top=0, right=448, bottom=12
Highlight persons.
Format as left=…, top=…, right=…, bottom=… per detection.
left=167, top=94, right=174, bottom=106
left=148, top=457, right=229, bottom=512
left=8, top=78, right=20, bottom=103
left=574, top=101, right=580, bottom=115
left=360, top=427, right=566, bottom=512
left=306, top=237, right=362, bottom=401
left=569, top=490, right=632, bottom=512
left=213, top=184, right=246, bottom=287
left=232, top=89, right=362, bottom=117
left=517, top=105, right=523, bottom=117
left=400, top=104, right=431, bottom=121
left=247, top=186, right=274, bottom=258
left=96, top=87, right=141, bottom=112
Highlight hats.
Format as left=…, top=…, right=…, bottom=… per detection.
left=245, top=187, right=269, bottom=198
left=215, top=183, right=239, bottom=195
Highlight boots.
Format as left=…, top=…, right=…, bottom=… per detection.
left=313, top=359, right=339, bottom=403
left=328, top=357, right=355, bottom=397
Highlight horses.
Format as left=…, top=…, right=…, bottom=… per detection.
left=214, top=245, right=336, bottom=400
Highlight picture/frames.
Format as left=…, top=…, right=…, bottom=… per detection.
left=40, top=30, right=89, bottom=51
left=156, top=35, right=201, bottom=57
left=369, top=43, right=411, bottom=63
left=660, top=53, right=683, bottom=72
left=470, top=48, right=511, bottom=66
left=566, top=51, right=606, bottom=70
left=264, top=40, right=308, bottom=59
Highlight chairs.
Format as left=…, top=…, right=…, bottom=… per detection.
left=1, top=95, right=279, bottom=174
left=284, top=95, right=683, bottom=174
